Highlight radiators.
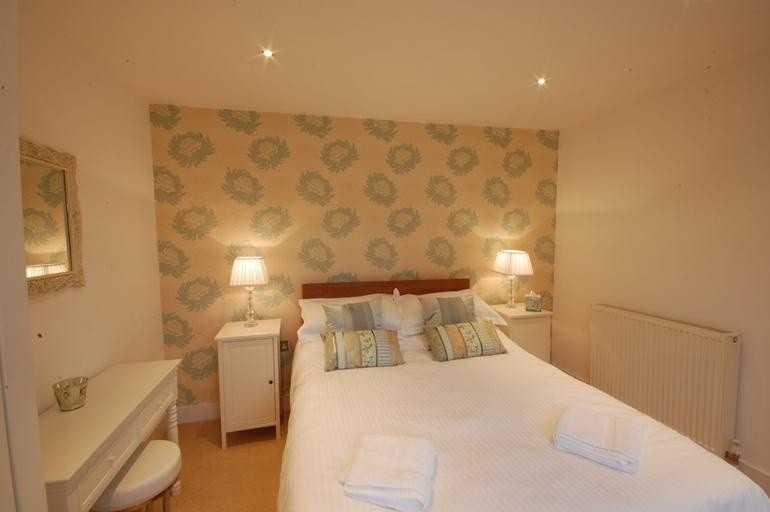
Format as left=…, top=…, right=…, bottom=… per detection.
left=590, top=304, right=741, bottom=466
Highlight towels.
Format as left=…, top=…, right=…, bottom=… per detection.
left=553, top=407, right=643, bottom=472
left=343, top=434, right=436, bottom=512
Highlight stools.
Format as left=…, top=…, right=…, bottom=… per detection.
left=90, top=440, right=182, bottom=512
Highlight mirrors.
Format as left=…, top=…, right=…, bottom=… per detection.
left=19, top=138, right=84, bottom=297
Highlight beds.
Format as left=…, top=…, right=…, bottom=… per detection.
left=278, top=278, right=770, bottom=512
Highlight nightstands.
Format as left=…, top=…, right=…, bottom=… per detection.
left=489, top=303, right=554, bottom=364
left=214, top=319, right=282, bottom=449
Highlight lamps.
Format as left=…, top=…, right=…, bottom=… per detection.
left=492, top=250, right=534, bottom=308
left=229, top=256, right=269, bottom=328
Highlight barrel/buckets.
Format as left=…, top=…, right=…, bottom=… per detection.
left=52, top=377, right=88, bottom=411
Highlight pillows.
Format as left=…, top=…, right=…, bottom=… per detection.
left=297, top=294, right=407, bottom=342
left=421, top=320, right=507, bottom=362
left=416, top=293, right=476, bottom=350
left=321, top=329, right=407, bottom=372
left=393, top=287, right=508, bottom=336
left=322, top=297, right=384, bottom=330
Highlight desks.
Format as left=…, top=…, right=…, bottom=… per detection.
left=37, top=359, right=184, bottom=512
